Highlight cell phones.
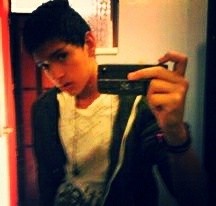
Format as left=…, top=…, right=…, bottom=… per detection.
left=97, top=64, right=168, bottom=95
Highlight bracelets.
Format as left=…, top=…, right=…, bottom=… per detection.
left=162, top=138, right=191, bottom=153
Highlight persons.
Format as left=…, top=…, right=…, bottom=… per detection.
left=23, top=0, right=208, bottom=206
